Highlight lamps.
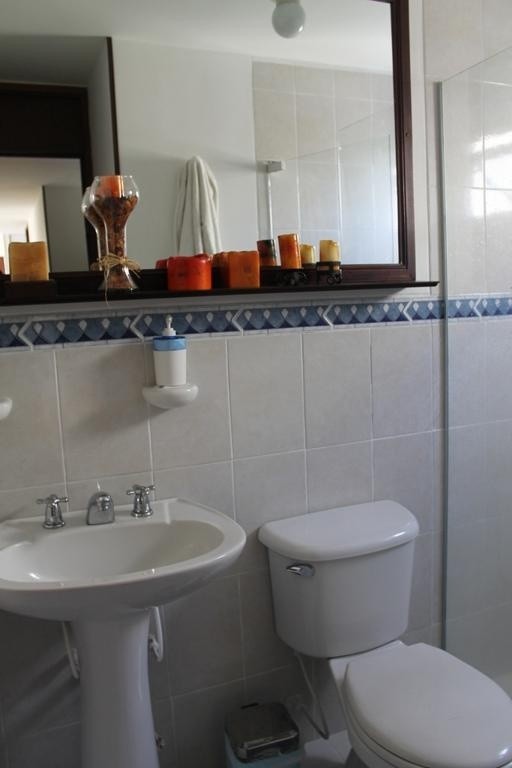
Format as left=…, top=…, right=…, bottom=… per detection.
left=271, top=0, right=307, bottom=40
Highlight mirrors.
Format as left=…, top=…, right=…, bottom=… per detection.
left=0, top=1, right=441, bottom=306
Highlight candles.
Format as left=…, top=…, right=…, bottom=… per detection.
left=299, top=244, right=315, bottom=263
left=320, top=239, right=340, bottom=263
left=256, top=239, right=277, bottom=267
left=278, top=233, right=302, bottom=269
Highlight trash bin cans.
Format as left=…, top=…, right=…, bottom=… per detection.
left=224, top=701, right=300, bottom=767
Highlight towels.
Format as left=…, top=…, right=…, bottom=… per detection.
left=175, top=154, right=222, bottom=257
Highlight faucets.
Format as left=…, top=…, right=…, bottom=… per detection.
left=86, top=491, right=116, bottom=525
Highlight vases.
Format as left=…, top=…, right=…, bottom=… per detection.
left=81, top=187, right=107, bottom=271
left=89, top=175, right=140, bottom=291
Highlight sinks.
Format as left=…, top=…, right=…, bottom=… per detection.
left=0, top=494, right=248, bottom=622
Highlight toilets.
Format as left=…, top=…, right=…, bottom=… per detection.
left=256, top=500, right=512, bottom=766
